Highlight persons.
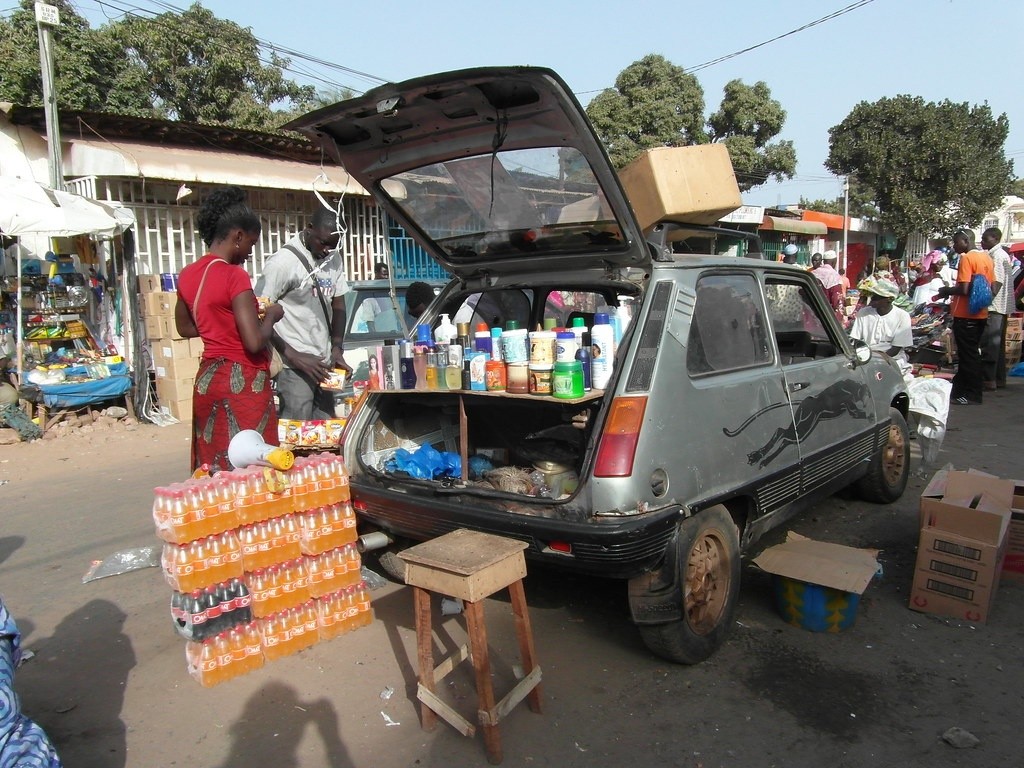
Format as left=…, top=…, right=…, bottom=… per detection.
left=175, top=186, right=285, bottom=477
left=362, top=263, right=402, bottom=333
left=406, top=282, right=490, bottom=341
left=765, top=244, right=918, bottom=438
left=908, top=228, right=1016, bottom=405
left=254, top=204, right=353, bottom=420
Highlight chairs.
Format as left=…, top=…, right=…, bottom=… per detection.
left=564, top=311, right=598, bottom=348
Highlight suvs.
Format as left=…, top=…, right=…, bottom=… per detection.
left=281, top=65, right=910, bottom=661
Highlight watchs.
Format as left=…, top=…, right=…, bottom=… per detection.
left=332, top=342, right=344, bottom=354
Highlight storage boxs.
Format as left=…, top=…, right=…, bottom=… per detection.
left=1004, top=318, right=1024, bottom=372
left=138, top=272, right=205, bottom=421
left=319, top=369, right=347, bottom=393
left=599, top=143, right=742, bottom=244
left=278, top=419, right=347, bottom=445
left=910, top=468, right=1024, bottom=626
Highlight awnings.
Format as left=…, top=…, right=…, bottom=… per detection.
left=41, top=136, right=407, bottom=200
left=758, top=215, right=827, bottom=234
left=0, top=174, right=135, bottom=384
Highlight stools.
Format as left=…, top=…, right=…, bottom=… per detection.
left=395, top=527, right=544, bottom=768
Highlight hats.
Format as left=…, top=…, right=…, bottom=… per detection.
left=822, top=250, right=837, bottom=260
left=868, top=279, right=899, bottom=298
left=784, top=244, right=798, bottom=254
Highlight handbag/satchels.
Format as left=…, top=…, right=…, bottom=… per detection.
left=269, top=341, right=283, bottom=378
left=968, top=273, right=992, bottom=314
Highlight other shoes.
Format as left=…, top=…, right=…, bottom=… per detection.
left=983, top=386, right=1006, bottom=390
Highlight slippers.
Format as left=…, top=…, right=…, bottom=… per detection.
left=952, top=397, right=982, bottom=405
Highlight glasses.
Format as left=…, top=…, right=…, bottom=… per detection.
left=408, top=312, right=420, bottom=320
left=954, top=229, right=970, bottom=239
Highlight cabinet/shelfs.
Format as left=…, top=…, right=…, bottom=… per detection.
left=20, top=308, right=91, bottom=363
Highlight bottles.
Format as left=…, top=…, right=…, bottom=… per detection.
left=152, top=452, right=373, bottom=687
left=413, top=313, right=614, bottom=398
